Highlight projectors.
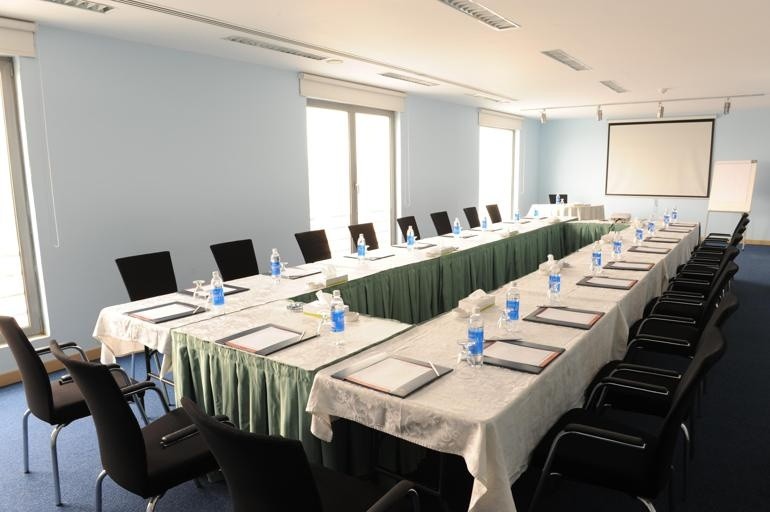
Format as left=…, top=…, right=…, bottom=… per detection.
left=608, top=213, right=631, bottom=223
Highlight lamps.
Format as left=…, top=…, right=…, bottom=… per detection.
left=540, top=110, right=548, bottom=123
left=656, top=101, right=664, bottom=119
left=724, top=97, right=731, bottom=115
left=596, top=106, right=602, bottom=121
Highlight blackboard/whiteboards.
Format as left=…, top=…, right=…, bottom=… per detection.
left=708, top=160, right=757, bottom=213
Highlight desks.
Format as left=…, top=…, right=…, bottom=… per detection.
left=525, top=202, right=605, bottom=221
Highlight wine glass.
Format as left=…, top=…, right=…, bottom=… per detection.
left=281, top=261, right=291, bottom=287
left=191, top=279, right=206, bottom=308
left=560, top=199, right=565, bottom=203
left=453, top=338, right=477, bottom=378
left=544, top=281, right=561, bottom=304
left=496, top=307, right=515, bottom=335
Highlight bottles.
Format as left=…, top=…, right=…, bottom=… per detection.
left=672, top=206, right=678, bottom=223
left=663, top=208, right=670, bottom=230
left=647, top=216, right=655, bottom=238
left=514, top=208, right=519, bottom=224
left=211, top=271, right=224, bottom=311
left=467, top=305, right=484, bottom=367
left=591, top=240, right=602, bottom=272
left=505, top=281, right=520, bottom=328
left=555, top=192, right=560, bottom=204
left=330, top=290, right=344, bottom=346
left=481, top=216, right=487, bottom=233
left=407, top=225, right=415, bottom=250
left=548, top=261, right=560, bottom=294
left=357, top=233, right=366, bottom=262
left=612, top=231, right=622, bottom=260
left=271, top=248, right=280, bottom=285
left=634, top=222, right=643, bottom=246
left=454, top=217, right=460, bottom=238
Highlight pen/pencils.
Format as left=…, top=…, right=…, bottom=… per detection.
left=300, top=330, right=305, bottom=339
left=428, top=361, right=439, bottom=376
left=193, top=306, right=200, bottom=313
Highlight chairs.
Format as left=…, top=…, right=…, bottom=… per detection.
left=430, top=211, right=452, bottom=236
left=462, top=206, right=481, bottom=228
left=48, top=340, right=236, bottom=512
left=0, top=316, right=149, bottom=505
left=486, top=203, right=501, bottom=224
left=548, top=193, right=567, bottom=204
